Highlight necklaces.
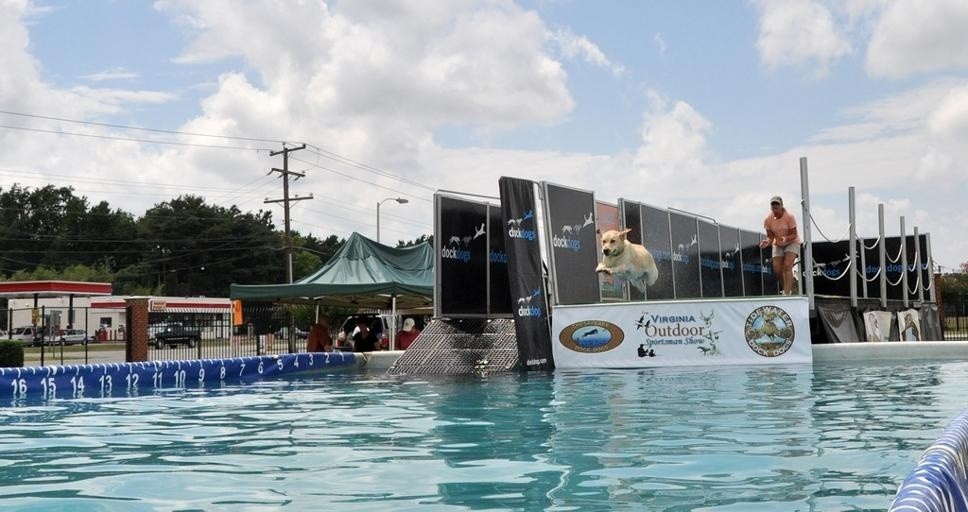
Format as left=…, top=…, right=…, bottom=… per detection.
left=773, top=214, right=778, bottom=220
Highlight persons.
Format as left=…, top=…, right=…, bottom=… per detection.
left=758, top=196, right=802, bottom=298
left=307, top=314, right=333, bottom=353
left=395, top=317, right=422, bottom=350
left=900, top=313, right=919, bottom=341
left=335, top=331, right=349, bottom=351
left=864, top=313, right=881, bottom=341
left=351, top=314, right=379, bottom=350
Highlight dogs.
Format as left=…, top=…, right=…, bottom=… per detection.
left=595, top=228, right=659, bottom=293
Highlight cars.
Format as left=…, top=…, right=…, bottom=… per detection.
left=274, top=327, right=310, bottom=339
left=0, top=325, right=88, bottom=346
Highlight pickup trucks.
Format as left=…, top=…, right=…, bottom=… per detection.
left=150, top=323, right=200, bottom=348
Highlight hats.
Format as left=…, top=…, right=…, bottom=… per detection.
left=770, top=196, right=783, bottom=206
left=403, top=318, right=415, bottom=332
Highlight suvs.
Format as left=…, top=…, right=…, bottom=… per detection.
left=335, top=313, right=401, bottom=350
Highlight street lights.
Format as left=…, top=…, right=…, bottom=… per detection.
left=376, top=196, right=409, bottom=242
